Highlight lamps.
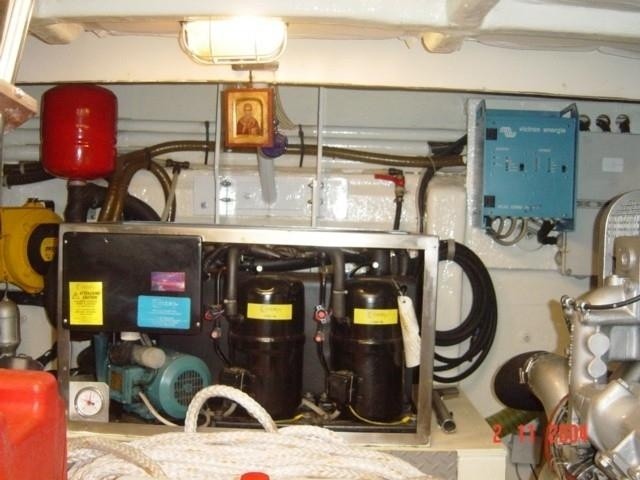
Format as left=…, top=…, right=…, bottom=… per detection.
left=178, top=17, right=291, bottom=68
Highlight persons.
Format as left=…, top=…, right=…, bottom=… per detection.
left=237, top=103, right=261, bottom=135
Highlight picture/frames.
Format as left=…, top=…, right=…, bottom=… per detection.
left=222, top=85, right=275, bottom=152
